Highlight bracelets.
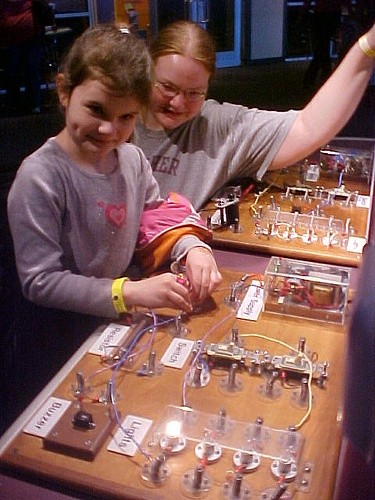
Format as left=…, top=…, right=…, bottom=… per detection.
left=358, top=34, right=375, bottom=57
left=111, top=277, right=134, bottom=314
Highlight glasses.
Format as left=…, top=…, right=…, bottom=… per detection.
left=153, top=81, right=206, bottom=102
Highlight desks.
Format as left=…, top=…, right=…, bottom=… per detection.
left=0, top=137, right=375, bottom=500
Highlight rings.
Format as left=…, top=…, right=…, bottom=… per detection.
left=177, top=273, right=187, bottom=285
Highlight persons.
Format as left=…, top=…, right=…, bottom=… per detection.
left=7, top=24, right=224, bottom=317
left=127, top=21, right=375, bottom=211
left=0, top=0, right=51, bottom=117
